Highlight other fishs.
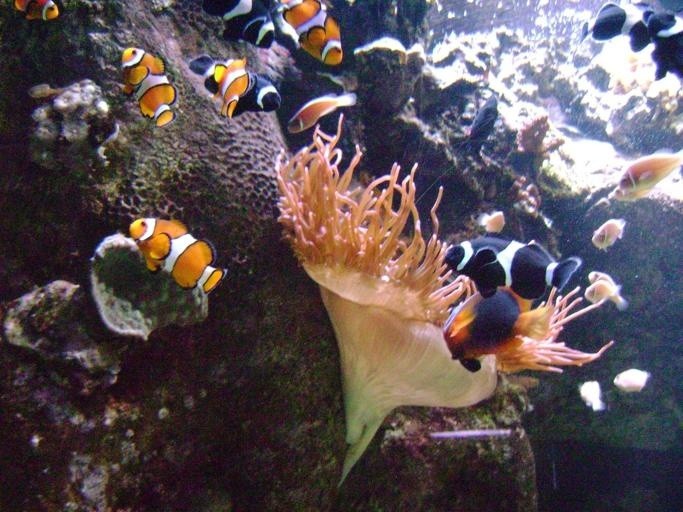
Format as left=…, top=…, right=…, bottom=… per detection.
left=440, top=208, right=651, bottom=412
